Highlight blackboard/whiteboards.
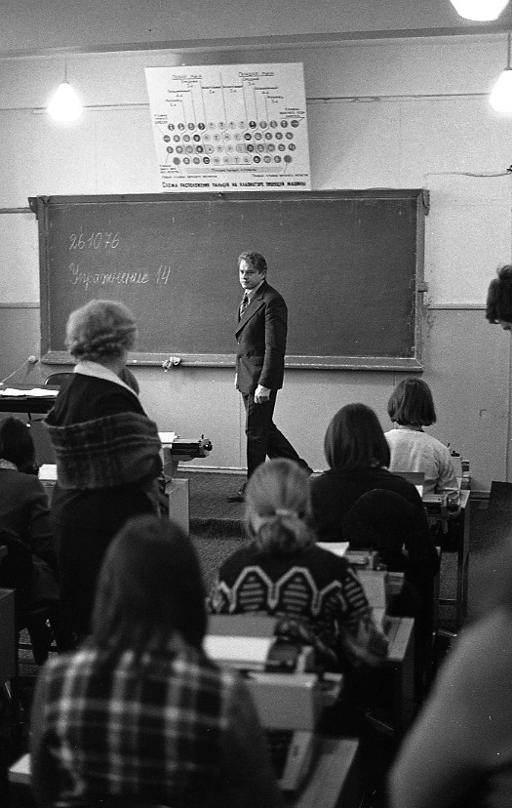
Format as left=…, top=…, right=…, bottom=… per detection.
left=35, top=189, right=430, bottom=373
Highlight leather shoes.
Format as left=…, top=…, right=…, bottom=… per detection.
left=227, top=482, right=248, bottom=501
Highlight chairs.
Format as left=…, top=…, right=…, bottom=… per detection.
left=26, top=372, right=75, bottom=421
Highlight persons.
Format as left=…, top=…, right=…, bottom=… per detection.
left=385, top=602, right=512, bottom=807
left=0, top=414, right=52, bottom=667
left=483, top=263, right=511, bottom=332
left=28, top=515, right=283, bottom=808
left=203, top=456, right=388, bottom=808
left=40, top=297, right=170, bottom=636
left=309, top=403, right=442, bottom=638
left=383, top=378, right=468, bottom=552
left=224, top=251, right=314, bottom=504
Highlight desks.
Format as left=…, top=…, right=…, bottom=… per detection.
left=0, top=382, right=62, bottom=414
left=0, top=488, right=469, bottom=805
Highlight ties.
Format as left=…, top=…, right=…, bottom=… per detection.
left=240, top=292, right=248, bottom=320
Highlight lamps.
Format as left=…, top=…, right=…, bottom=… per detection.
left=489, top=26, right=512, bottom=113
left=449, top=0, right=509, bottom=22
left=45, top=46, right=85, bottom=122
